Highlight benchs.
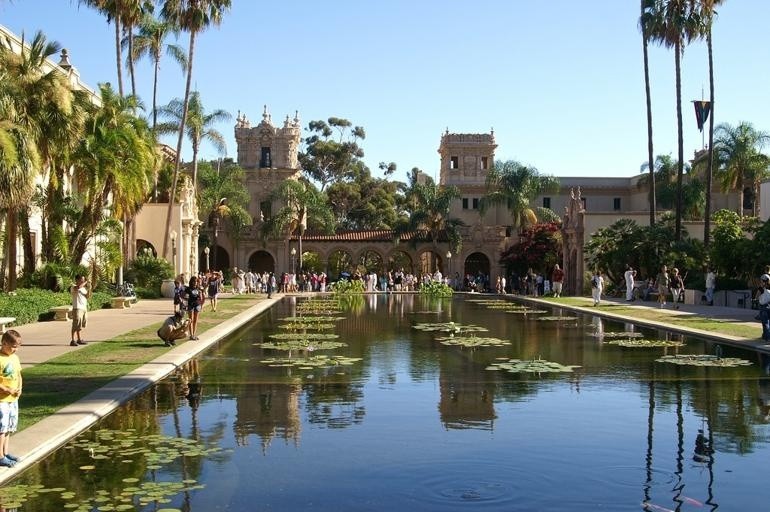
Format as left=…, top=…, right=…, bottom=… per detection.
left=649, top=292, right=671, bottom=303
left=50, top=305, right=74, bottom=321
left=0, top=317, right=16, bottom=335
left=110, top=296, right=136, bottom=309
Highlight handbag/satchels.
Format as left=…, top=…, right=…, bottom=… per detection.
left=590, top=280, right=598, bottom=289
left=758, top=288, right=770, bottom=306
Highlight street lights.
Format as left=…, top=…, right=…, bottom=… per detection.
left=290, top=246, right=297, bottom=274
left=169, top=229, right=177, bottom=280
left=442, top=250, right=453, bottom=280
left=203, top=246, right=212, bottom=270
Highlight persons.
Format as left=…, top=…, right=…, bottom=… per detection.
left=70, top=275, right=91, bottom=346
left=188, top=359, right=201, bottom=411
left=755, top=273, right=770, bottom=345
left=705, top=267, right=715, bottom=305
left=1, top=330, right=22, bottom=467
left=176, top=368, right=182, bottom=398
left=158, top=270, right=224, bottom=348
left=183, top=366, right=190, bottom=398
left=755, top=265, right=770, bottom=320
left=591, top=265, right=684, bottom=310
left=220, top=287, right=235, bottom=295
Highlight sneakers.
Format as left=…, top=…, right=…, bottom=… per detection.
left=165, top=341, right=177, bottom=347
left=69, top=340, right=79, bottom=346
left=0, top=457, right=16, bottom=468
left=4, top=453, right=20, bottom=462
left=189, top=335, right=201, bottom=340
left=77, top=339, right=88, bottom=345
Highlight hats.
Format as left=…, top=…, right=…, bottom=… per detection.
left=759, top=274, right=770, bottom=281
